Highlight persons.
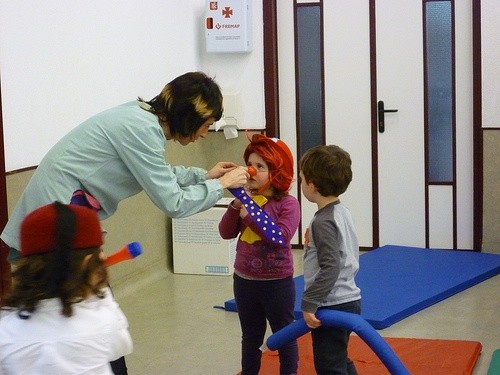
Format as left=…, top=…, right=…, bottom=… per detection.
left=218, top=134, right=300, bottom=375
left=0, top=71, right=250, bottom=375
left=298, top=144, right=362, bottom=375
left=0, top=201, right=132, bottom=375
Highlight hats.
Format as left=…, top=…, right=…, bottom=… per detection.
left=252, top=134, right=294, bottom=192
left=20, top=201, right=104, bottom=254
